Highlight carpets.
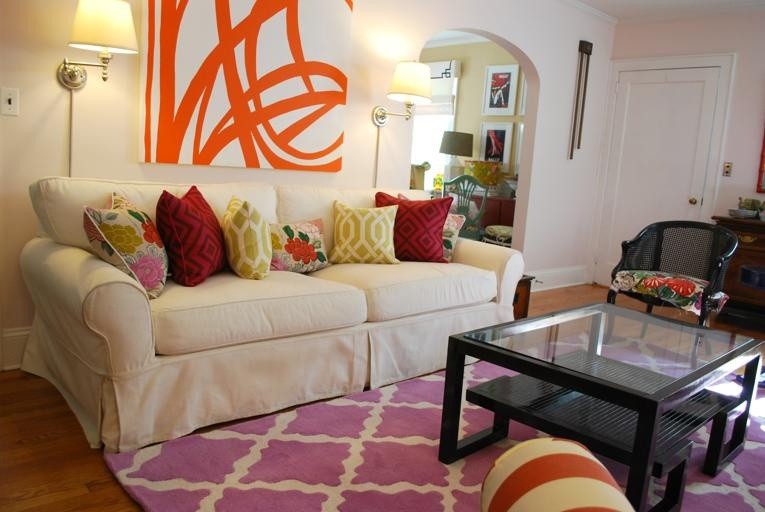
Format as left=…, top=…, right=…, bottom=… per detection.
left=100, top=337, right=765, bottom=510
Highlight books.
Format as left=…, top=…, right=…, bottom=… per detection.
left=410, top=164, right=425, bottom=189
left=461, top=183, right=511, bottom=198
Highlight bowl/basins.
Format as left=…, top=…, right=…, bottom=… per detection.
left=728, top=208, right=757, bottom=219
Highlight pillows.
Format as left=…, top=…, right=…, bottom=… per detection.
left=330, top=199, right=402, bottom=266
left=375, top=189, right=452, bottom=263
left=220, top=193, right=272, bottom=281
left=83, top=190, right=168, bottom=299
left=271, top=217, right=332, bottom=274
left=399, top=193, right=467, bottom=262
left=156, top=186, right=225, bottom=288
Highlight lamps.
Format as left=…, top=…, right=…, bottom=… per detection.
left=58, top=0, right=138, bottom=90
left=372, top=60, right=432, bottom=127
left=439, top=131, right=473, bottom=190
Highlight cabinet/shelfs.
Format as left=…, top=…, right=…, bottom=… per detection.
left=469, top=195, right=515, bottom=226
left=711, top=215, right=765, bottom=332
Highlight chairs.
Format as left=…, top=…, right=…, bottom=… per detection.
left=604, top=220, right=740, bottom=370
left=441, top=174, right=489, bottom=241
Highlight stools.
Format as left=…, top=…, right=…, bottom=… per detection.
left=482, top=224, right=513, bottom=247
left=479, top=436, right=635, bottom=511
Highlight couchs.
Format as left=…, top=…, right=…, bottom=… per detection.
left=16, top=177, right=524, bottom=455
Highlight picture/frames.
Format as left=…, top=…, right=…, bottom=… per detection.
left=482, top=64, right=519, bottom=116
left=515, top=122, right=524, bottom=173
left=520, top=72, right=527, bottom=116
left=479, top=122, right=514, bottom=174
